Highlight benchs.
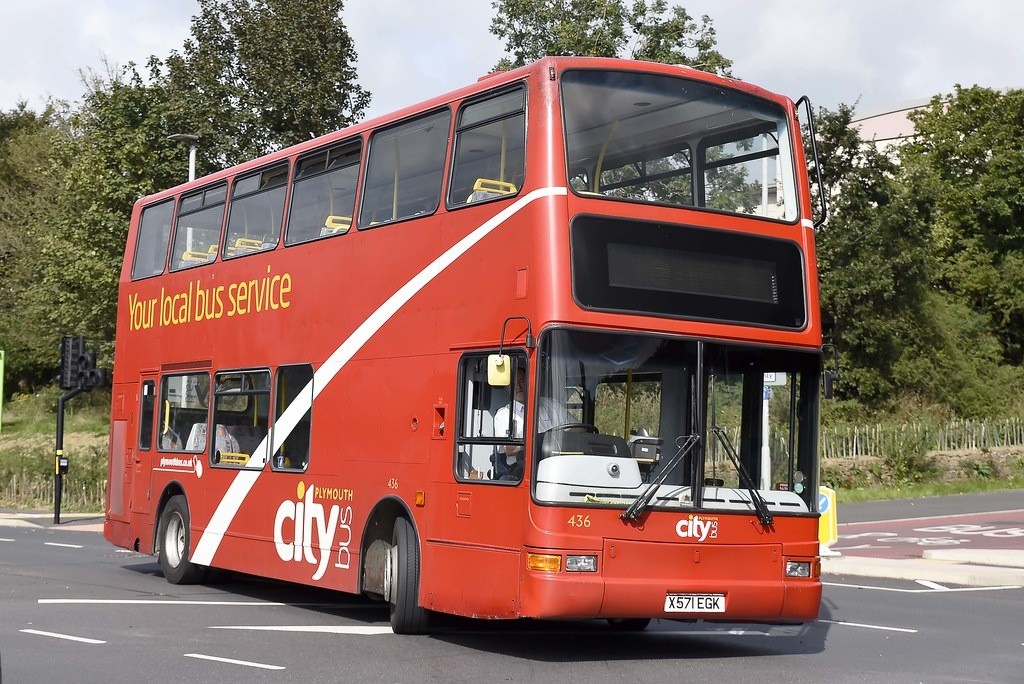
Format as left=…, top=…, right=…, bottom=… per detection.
left=186, top=422, right=266, bottom=464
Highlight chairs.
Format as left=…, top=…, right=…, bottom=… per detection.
left=467, top=178, right=517, bottom=204
left=178, top=238, right=264, bottom=268
left=319, top=215, right=353, bottom=235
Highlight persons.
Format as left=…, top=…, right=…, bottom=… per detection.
left=492, top=367, right=588, bottom=478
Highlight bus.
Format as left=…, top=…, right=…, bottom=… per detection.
left=99, top=56, right=841, bottom=636
